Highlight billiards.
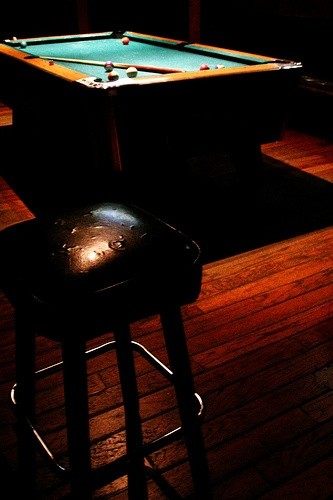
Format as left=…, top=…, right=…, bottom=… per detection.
left=121, top=37, right=130, bottom=45
left=103, top=61, right=114, bottom=72
left=126, top=67, right=137, bottom=78
left=19, top=40, right=27, bottom=48
left=199, top=64, right=210, bottom=71
left=107, top=71, right=119, bottom=81
left=214, top=64, right=225, bottom=69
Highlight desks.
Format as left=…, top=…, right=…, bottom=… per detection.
left=0, top=30, right=304, bottom=207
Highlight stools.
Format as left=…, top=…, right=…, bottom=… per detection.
left=0, top=199, right=215, bottom=500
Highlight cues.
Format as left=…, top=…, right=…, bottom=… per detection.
left=40, top=55, right=186, bottom=74
